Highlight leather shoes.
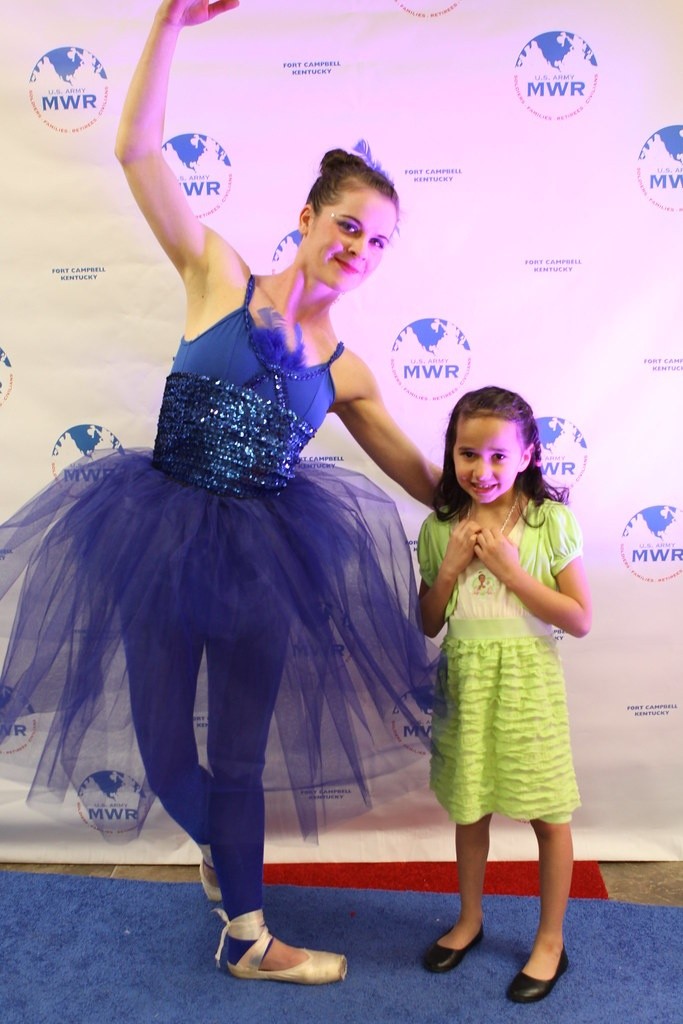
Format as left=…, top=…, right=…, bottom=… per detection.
left=506, top=943, right=569, bottom=1003
left=422, top=922, right=483, bottom=973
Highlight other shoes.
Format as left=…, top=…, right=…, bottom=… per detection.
left=226, top=926, right=348, bottom=985
left=199, top=857, right=222, bottom=901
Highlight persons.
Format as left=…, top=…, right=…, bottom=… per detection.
left=415, top=387, right=592, bottom=1004
left=0, top=0, right=443, bottom=985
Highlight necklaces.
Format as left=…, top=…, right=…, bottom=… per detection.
left=467, top=491, right=520, bottom=534
